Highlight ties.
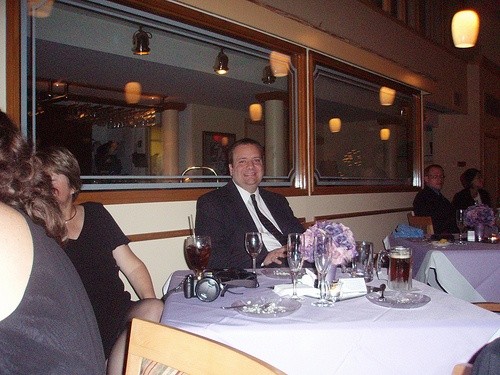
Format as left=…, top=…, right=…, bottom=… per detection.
left=250, top=194, right=288, bottom=246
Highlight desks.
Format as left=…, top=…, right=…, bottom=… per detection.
left=384, top=235, right=500, bottom=313
left=161, top=268, right=500, bottom=374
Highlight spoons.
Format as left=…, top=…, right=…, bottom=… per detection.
left=378, top=283, right=386, bottom=301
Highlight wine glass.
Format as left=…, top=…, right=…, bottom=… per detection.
left=245, top=232, right=262, bottom=275
left=286, top=233, right=305, bottom=301
left=454, top=209, right=468, bottom=245
left=312, top=236, right=333, bottom=307
left=184, top=236, right=211, bottom=278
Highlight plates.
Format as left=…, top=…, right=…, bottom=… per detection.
left=365, top=290, right=431, bottom=309
left=230, top=296, right=302, bottom=318
left=261, top=268, right=305, bottom=280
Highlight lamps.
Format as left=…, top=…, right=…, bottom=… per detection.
left=329, top=86, right=396, bottom=141
left=132, top=25, right=152, bottom=54
left=249, top=104, right=262, bottom=121
left=262, top=51, right=290, bottom=85
left=125, top=82, right=141, bottom=104
left=213, top=48, right=228, bottom=75
left=451, top=9, right=480, bottom=49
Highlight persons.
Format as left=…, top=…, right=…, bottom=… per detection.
left=37, top=146, right=164, bottom=375
left=195, top=138, right=316, bottom=269
left=0, top=110, right=101, bottom=375
left=413, top=165, right=493, bottom=236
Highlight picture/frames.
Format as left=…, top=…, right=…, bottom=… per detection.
left=202, top=131, right=236, bottom=182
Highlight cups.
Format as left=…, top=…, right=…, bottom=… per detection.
left=351, top=240, right=374, bottom=281
left=377, top=246, right=413, bottom=290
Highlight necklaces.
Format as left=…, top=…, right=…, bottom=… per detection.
left=64, top=205, right=77, bottom=224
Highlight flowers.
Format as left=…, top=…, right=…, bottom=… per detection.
left=299, top=219, right=359, bottom=265
left=464, top=204, right=495, bottom=227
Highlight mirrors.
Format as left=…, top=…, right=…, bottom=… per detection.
left=308, top=50, right=422, bottom=195
left=6, top=0, right=307, bottom=205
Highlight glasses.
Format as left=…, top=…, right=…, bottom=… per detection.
left=426, top=175, right=446, bottom=179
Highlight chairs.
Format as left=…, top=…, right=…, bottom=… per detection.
left=125, top=318, right=286, bottom=375
left=408, top=212, right=434, bottom=235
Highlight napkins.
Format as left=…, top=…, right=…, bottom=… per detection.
left=273, top=268, right=367, bottom=301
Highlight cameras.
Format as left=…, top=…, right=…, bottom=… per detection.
left=182, top=272, right=228, bottom=303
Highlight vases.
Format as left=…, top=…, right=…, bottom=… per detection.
left=476, top=224, right=484, bottom=241
left=318, top=261, right=337, bottom=282
